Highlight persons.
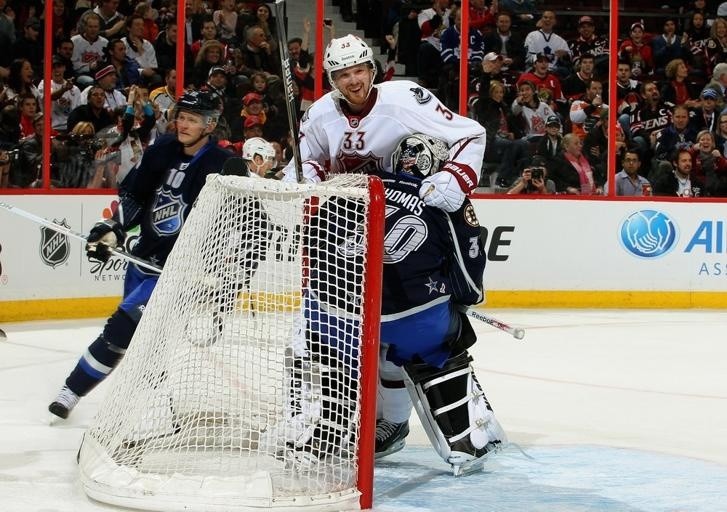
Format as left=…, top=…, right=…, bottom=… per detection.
left=50, top=88, right=270, bottom=420
left=281, top=33, right=487, bottom=446
left=293, top=134, right=506, bottom=475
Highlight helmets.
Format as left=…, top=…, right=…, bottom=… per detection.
left=174, top=90, right=224, bottom=137
left=324, top=35, right=377, bottom=89
left=243, top=136, right=276, bottom=179
left=390, top=134, right=449, bottom=177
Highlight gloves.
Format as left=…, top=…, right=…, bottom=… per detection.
left=418, top=171, right=465, bottom=213
left=87, top=218, right=124, bottom=261
left=282, top=160, right=326, bottom=184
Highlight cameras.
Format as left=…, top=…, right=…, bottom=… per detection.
left=530, top=168, right=543, bottom=178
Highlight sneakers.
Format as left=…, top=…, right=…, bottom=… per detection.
left=374, top=419, right=408, bottom=454
left=49, top=386, right=80, bottom=419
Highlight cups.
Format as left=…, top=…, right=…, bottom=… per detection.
left=643, top=183, right=652, bottom=197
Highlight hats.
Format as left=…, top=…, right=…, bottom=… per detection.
left=483, top=51, right=502, bottom=64
left=208, top=66, right=224, bottom=77
left=94, top=60, right=115, bottom=80
left=702, top=89, right=718, bottom=99
left=244, top=116, right=263, bottom=128
left=534, top=52, right=550, bottom=62
left=243, top=93, right=262, bottom=105
left=545, top=116, right=561, bottom=127
